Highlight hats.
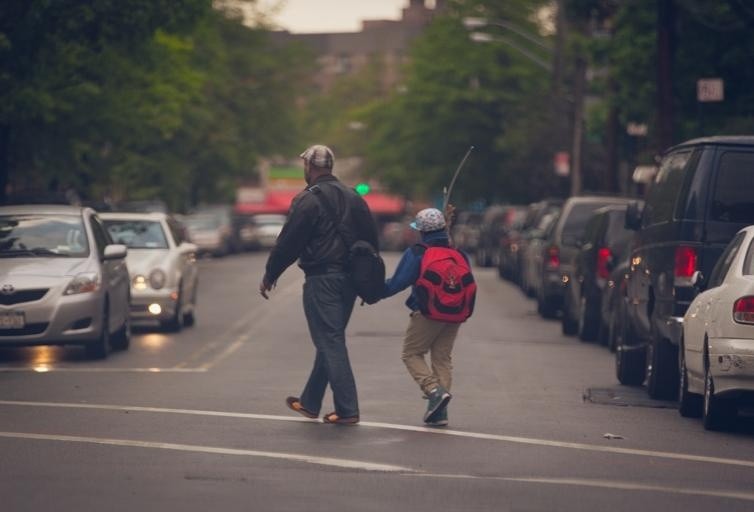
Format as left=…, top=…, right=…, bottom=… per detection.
left=409, top=207, right=447, bottom=233
left=299, top=143, right=335, bottom=170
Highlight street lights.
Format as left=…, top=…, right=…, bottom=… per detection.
left=460, top=12, right=582, bottom=197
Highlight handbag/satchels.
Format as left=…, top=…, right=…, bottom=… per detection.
left=345, top=241, right=388, bottom=306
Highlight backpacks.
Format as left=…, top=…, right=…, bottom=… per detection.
left=413, top=241, right=477, bottom=324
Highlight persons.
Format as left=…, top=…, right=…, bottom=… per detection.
left=383, top=208, right=468, bottom=427
left=259, top=144, right=376, bottom=425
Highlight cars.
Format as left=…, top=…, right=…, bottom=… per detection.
left=92, top=208, right=199, bottom=333
left=0, top=202, right=135, bottom=363
left=675, top=220, right=754, bottom=435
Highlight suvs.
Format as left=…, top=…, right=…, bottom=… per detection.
left=609, top=130, right=754, bottom=397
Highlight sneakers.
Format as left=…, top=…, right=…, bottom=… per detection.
left=431, top=407, right=449, bottom=426
left=285, top=393, right=321, bottom=420
left=422, top=387, right=452, bottom=424
left=323, top=409, right=360, bottom=424
